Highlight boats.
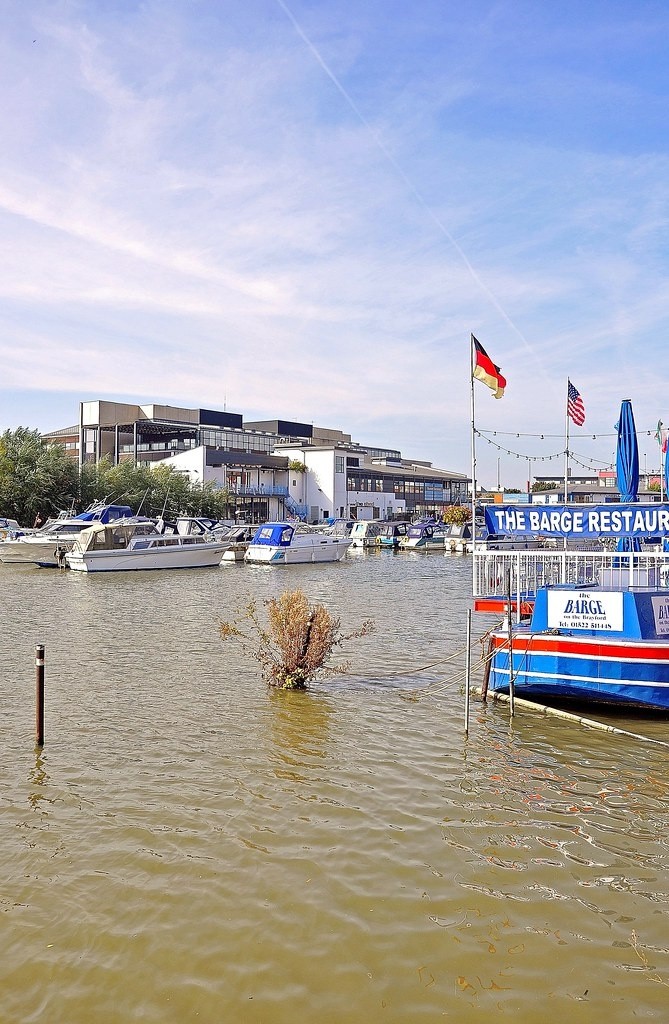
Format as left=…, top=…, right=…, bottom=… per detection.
left=469, top=331, right=669, bottom=712
left=0, top=487, right=548, bottom=572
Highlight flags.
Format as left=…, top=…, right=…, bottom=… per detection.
left=566, top=381, right=585, bottom=427
left=613, top=418, right=668, bottom=453
left=471, top=334, right=507, bottom=400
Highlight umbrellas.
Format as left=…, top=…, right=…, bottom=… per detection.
left=607, top=401, right=644, bottom=569
left=660, top=427, right=669, bottom=562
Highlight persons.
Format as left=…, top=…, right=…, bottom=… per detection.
left=231, top=478, right=301, bottom=523
